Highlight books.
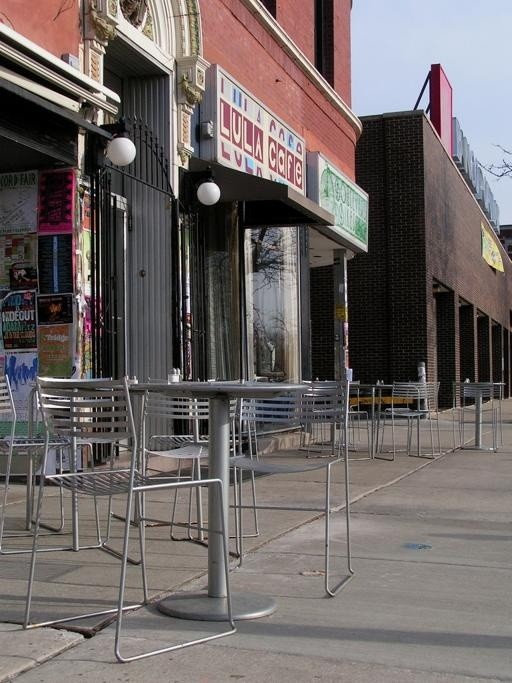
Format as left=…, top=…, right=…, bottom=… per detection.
left=0, top=168, right=105, bottom=421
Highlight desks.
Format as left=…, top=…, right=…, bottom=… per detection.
left=155, top=378, right=312, bottom=622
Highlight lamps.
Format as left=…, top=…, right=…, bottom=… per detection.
left=107, top=128, right=137, bottom=167
left=196, top=167, right=220, bottom=206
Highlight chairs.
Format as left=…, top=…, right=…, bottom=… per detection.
left=202, top=370, right=358, bottom=600
left=2, top=373, right=103, bottom=559
left=20, top=375, right=242, bottom=663
left=147, top=374, right=264, bottom=547
left=298, top=367, right=503, bottom=470
